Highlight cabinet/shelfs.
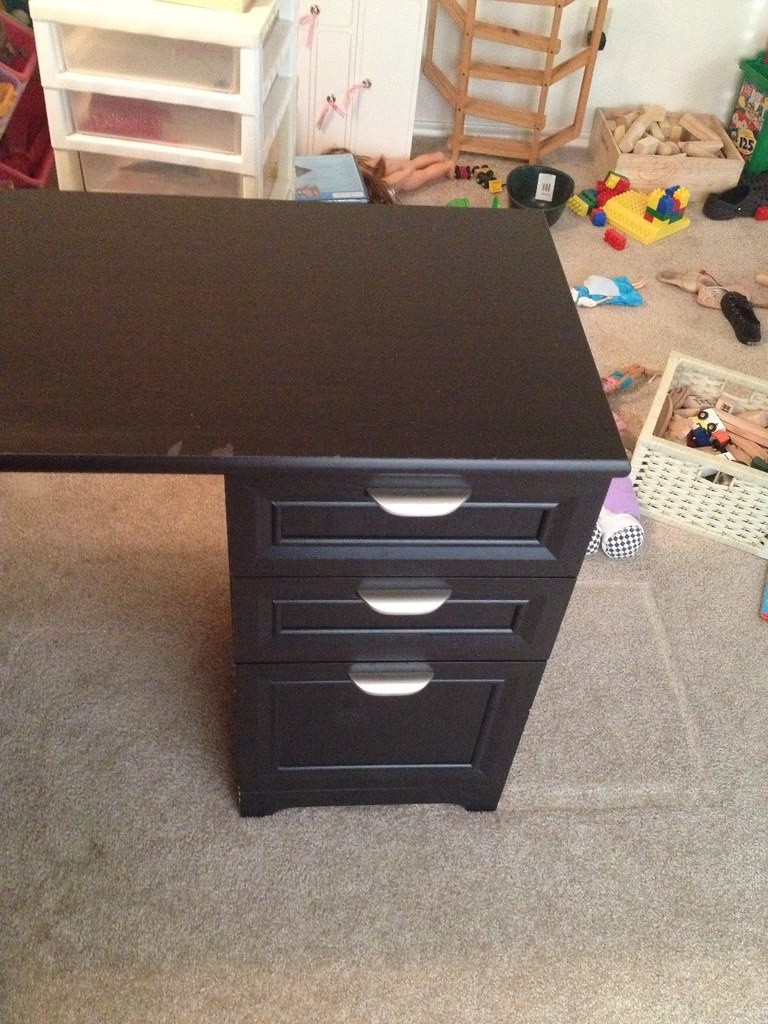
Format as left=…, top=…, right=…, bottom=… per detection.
left=224, top=473, right=615, bottom=819
left=26, top=0, right=297, bottom=203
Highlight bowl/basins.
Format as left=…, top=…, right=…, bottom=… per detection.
left=506, top=165, right=575, bottom=227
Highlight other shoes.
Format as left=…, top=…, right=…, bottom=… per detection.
left=657, top=270, right=717, bottom=294
left=697, top=286, right=750, bottom=309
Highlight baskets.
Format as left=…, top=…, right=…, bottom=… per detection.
left=629, top=349, right=768, bottom=557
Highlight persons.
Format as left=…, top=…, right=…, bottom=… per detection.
left=601, top=363, right=660, bottom=394
left=571, top=275, right=648, bottom=307
left=320, top=148, right=455, bottom=205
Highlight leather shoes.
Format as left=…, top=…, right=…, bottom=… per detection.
left=703, top=182, right=768, bottom=220
left=720, top=291, right=761, bottom=348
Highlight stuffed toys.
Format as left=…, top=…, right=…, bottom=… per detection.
left=584, top=475, right=644, bottom=558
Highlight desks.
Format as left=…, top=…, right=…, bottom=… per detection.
left=0, top=191, right=640, bottom=819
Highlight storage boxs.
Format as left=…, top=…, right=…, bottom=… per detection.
left=626, top=351, right=767, bottom=558
left=0, top=10, right=53, bottom=192
left=295, top=154, right=369, bottom=204
left=587, top=106, right=744, bottom=202
